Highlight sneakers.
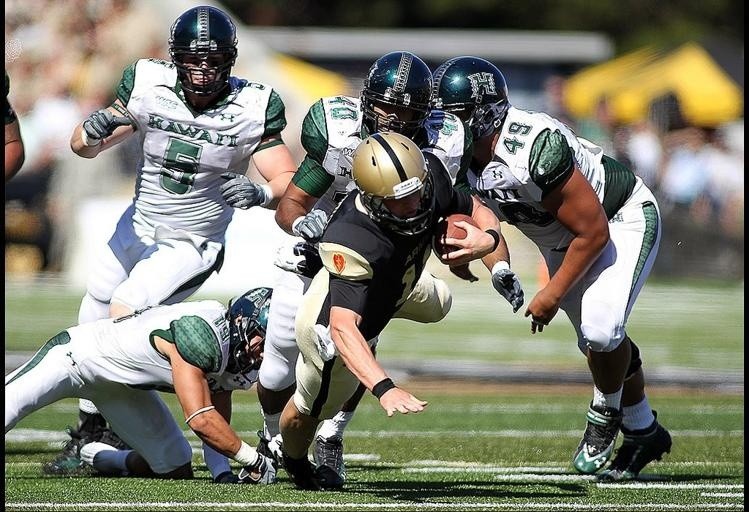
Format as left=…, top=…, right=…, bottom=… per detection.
left=314, top=439, right=345, bottom=490
left=44, top=426, right=108, bottom=476
left=81, top=443, right=119, bottom=476
left=574, top=402, right=622, bottom=475
left=270, top=436, right=316, bottom=491
left=599, top=416, right=672, bottom=483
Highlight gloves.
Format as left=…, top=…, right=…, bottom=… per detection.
left=219, top=173, right=265, bottom=208
left=492, top=263, right=524, bottom=313
left=243, top=452, right=278, bottom=485
left=82, top=110, right=130, bottom=146
left=292, top=209, right=327, bottom=239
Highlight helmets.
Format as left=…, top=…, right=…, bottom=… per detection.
left=169, top=5, right=238, bottom=95
left=353, top=133, right=434, bottom=236
left=431, top=56, right=510, bottom=139
left=228, top=286, right=274, bottom=387
left=362, top=51, right=432, bottom=138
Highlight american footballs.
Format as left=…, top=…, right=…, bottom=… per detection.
left=432, top=213, right=482, bottom=264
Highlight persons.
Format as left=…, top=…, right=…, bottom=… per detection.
left=0, top=70, right=26, bottom=183
left=238, top=52, right=523, bottom=482
left=44, top=6, right=294, bottom=477
left=2, top=289, right=276, bottom=485
left=279, top=134, right=501, bottom=494
left=430, top=54, right=671, bottom=482
